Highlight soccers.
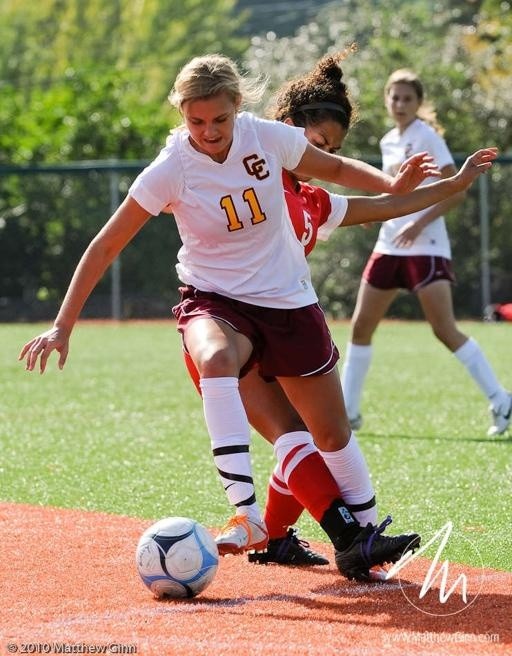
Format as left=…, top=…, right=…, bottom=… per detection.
left=136, top=517, right=218, bottom=598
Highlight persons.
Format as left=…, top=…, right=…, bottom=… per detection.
left=16, top=52, right=443, bottom=583
left=182, top=43, right=499, bottom=579
left=341, top=67, right=512, bottom=439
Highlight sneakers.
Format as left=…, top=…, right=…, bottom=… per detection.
left=485, top=389, right=511, bottom=438
left=333, top=514, right=423, bottom=583
left=213, top=512, right=271, bottom=557
left=348, top=414, right=364, bottom=432
left=247, top=527, right=330, bottom=567
